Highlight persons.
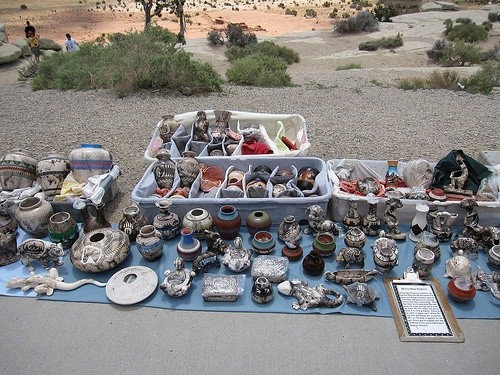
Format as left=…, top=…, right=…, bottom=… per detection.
left=28, top=31, right=41, bottom=63
left=64, top=33, right=80, bottom=53
left=25, top=21, right=35, bottom=38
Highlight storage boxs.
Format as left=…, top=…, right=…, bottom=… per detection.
left=131, top=156, right=332, bottom=224
left=326, top=158, right=500, bottom=225
left=143, top=110, right=311, bottom=166
